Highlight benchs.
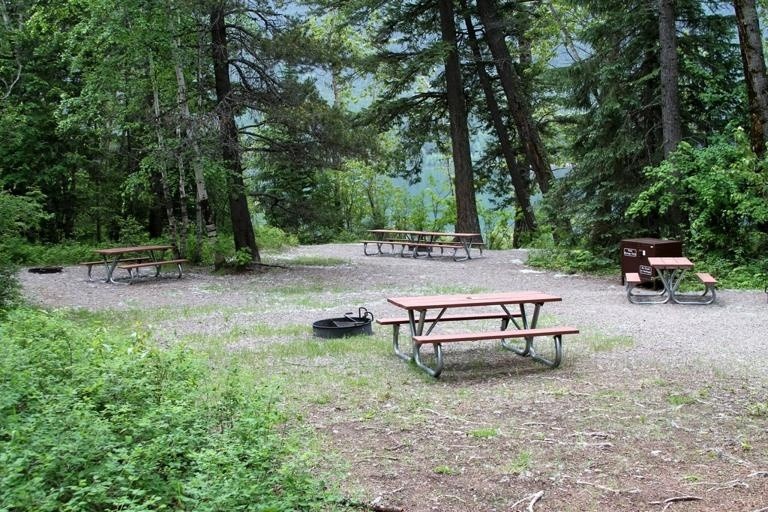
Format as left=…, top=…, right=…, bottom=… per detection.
left=361, top=238, right=486, bottom=262
left=375, top=313, right=581, bottom=378
left=694, top=273, right=718, bottom=304
left=79, top=256, right=189, bottom=286
left=624, top=272, right=641, bottom=304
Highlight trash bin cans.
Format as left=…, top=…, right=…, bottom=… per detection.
left=621, top=238, right=682, bottom=286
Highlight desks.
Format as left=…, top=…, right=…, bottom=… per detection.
left=646, top=256, right=694, bottom=305
left=91, top=245, right=176, bottom=285
left=387, top=291, right=562, bottom=377
left=367, top=229, right=482, bottom=262
